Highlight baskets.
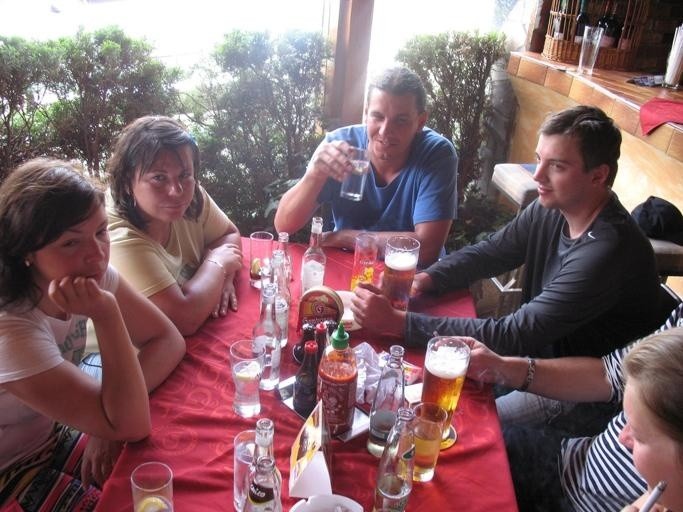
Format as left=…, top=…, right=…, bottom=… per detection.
left=543, top=0, right=652, bottom=73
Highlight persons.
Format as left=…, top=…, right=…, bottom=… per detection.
left=83, top=114, right=244, bottom=355
left=618, top=326, right=682, bottom=512
left=433, top=303, right=683, bottom=512
left=349, top=105, right=658, bottom=440
left=274, top=68, right=459, bottom=268
left=0, top=157, right=186, bottom=511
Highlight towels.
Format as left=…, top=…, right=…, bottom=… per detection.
left=639, top=88, right=683, bottom=138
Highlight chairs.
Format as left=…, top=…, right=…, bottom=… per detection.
left=547, top=282, right=681, bottom=440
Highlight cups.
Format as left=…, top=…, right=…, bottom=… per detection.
left=130, top=462, right=174, bottom=512
left=350, top=234, right=377, bottom=293
left=340, top=147, right=372, bottom=202
left=230, top=340, right=266, bottom=418
left=234, top=430, right=255, bottom=512
left=414, top=336, right=471, bottom=451
left=578, top=26, right=604, bottom=77
left=384, top=236, right=420, bottom=313
left=250, top=231, right=274, bottom=289
left=663, top=28, right=683, bottom=89
left=408, top=402, right=448, bottom=481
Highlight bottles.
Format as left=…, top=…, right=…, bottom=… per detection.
left=293, top=341, right=319, bottom=416
left=273, top=249, right=291, bottom=349
left=319, top=322, right=358, bottom=436
left=280, top=232, right=291, bottom=280
left=301, top=218, right=327, bottom=297
left=619, top=5, right=634, bottom=51
left=243, top=453, right=276, bottom=512
left=253, top=285, right=281, bottom=390
left=253, top=419, right=280, bottom=509
left=599, top=4, right=625, bottom=48
left=258, top=262, right=273, bottom=301
left=574, top=0, right=590, bottom=44
left=596, top=0, right=613, bottom=28
left=367, top=346, right=404, bottom=458
left=552, top=0, right=575, bottom=41
left=374, top=408, right=414, bottom=512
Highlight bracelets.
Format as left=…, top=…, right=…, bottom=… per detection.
left=204, top=258, right=227, bottom=278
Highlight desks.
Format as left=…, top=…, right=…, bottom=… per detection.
left=92, top=234, right=518, bottom=512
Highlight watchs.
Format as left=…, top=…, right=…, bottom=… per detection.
left=517, top=356, right=536, bottom=392
